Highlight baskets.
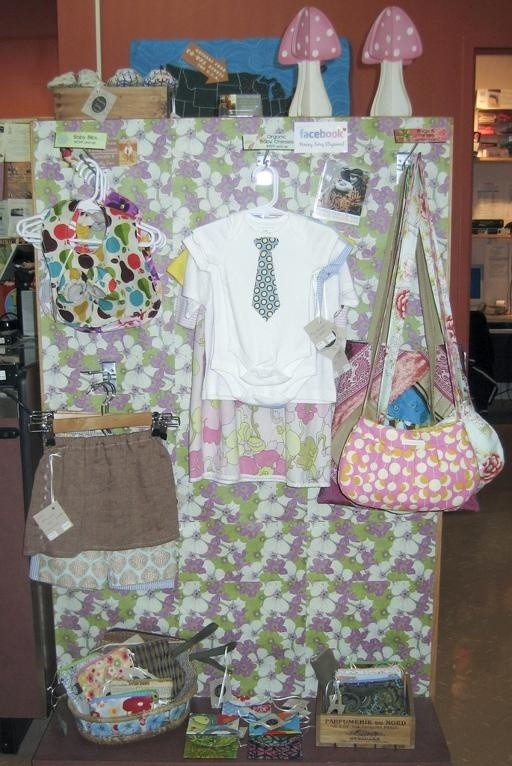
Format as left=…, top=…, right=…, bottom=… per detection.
left=67, top=628, right=198, bottom=746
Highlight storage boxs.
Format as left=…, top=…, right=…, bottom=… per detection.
left=53, top=85, right=169, bottom=119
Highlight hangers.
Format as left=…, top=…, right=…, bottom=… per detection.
left=15, top=156, right=167, bottom=253
left=184, top=161, right=339, bottom=243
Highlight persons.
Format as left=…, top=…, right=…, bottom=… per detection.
left=334, top=167, right=366, bottom=202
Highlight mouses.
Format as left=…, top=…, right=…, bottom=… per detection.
left=495, top=307, right=508, bottom=314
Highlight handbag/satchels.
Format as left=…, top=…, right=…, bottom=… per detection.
left=317, top=338, right=505, bottom=514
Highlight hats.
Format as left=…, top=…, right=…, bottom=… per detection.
left=340, top=168, right=363, bottom=181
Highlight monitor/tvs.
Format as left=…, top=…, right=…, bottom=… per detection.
left=470, top=263, right=485, bottom=311
